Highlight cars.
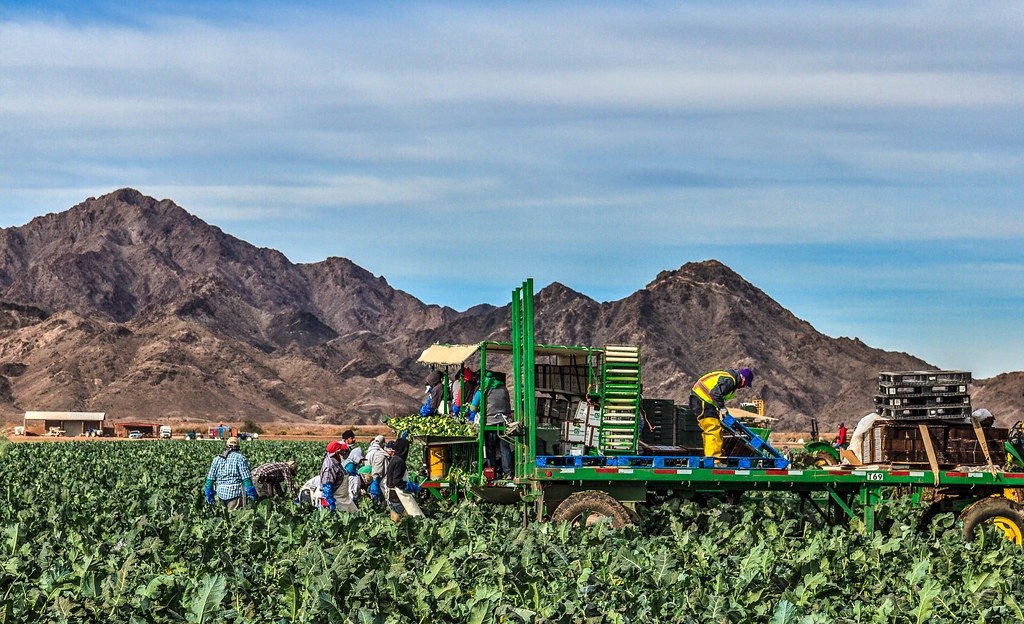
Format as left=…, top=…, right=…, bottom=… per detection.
left=129, top=431, right=142, bottom=439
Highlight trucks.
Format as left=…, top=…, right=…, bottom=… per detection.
left=161, top=426, right=172, bottom=439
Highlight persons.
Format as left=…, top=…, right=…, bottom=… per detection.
left=782, top=446, right=793, bottom=462
left=420, top=367, right=512, bottom=480
left=204, top=437, right=260, bottom=511
left=834, top=422, right=848, bottom=449
left=251, top=460, right=297, bottom=499
left=298, top=429, right=420, bottom=522
left=689, top=368, right=753, bottom=467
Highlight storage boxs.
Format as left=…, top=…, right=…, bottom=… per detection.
left=642, top=399, right=771, bottom=466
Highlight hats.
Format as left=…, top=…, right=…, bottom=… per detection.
left=285, top=460, right=297, bottom=476
left=740, top=367, right=756, bottom=390
left=360, top=472, right=373, bottom=494
left=384, top=440, right=397, bottom=452
left=340, top=429, right=356, bottom=439
left=326, top=441, right=349, bottom=458
left=225, top=436, right=240, bottom=447
left=838, top=421, right=844, bottom=427
left=374, top=434, right=385, bottom=445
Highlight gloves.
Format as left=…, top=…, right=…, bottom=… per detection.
left=247, top=487, right=260, bottom=502
left=367, top=476, right=383, bottom=504
left=203, top=493, right=218, bottom=506
left=719, top=405, right=729, bottom=418
left=322, top=483, right=336, bottom=513
left=405, top=481, right=423, bottom=493
